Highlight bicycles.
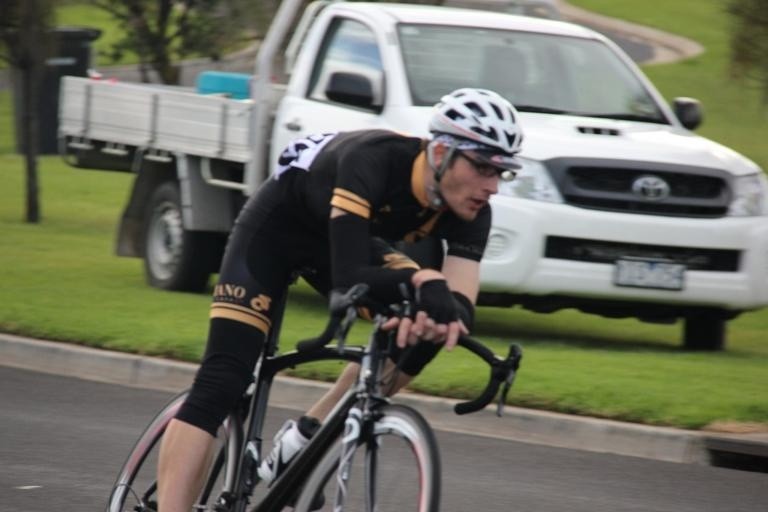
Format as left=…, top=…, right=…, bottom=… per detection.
left=101, top=275, right=527, bottom=512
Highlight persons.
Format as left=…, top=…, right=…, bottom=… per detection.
left=152, top=89, right=526, bottom=511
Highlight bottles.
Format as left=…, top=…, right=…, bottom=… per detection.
left=256, top=414, right=321, bottom=487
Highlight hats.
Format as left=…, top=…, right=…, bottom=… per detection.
left=433, top=134, right=521, bottom=169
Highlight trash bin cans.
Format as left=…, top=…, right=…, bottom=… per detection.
left=0, top=26, right=101, bottom=156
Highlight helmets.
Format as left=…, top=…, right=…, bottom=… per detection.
left=428, top=87, right=522, bottom=153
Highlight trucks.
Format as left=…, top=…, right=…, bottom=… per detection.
left=53, top=0, right=768, bottom=358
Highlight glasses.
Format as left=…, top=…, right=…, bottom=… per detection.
left=458, top=149, right=516, bottom=182
